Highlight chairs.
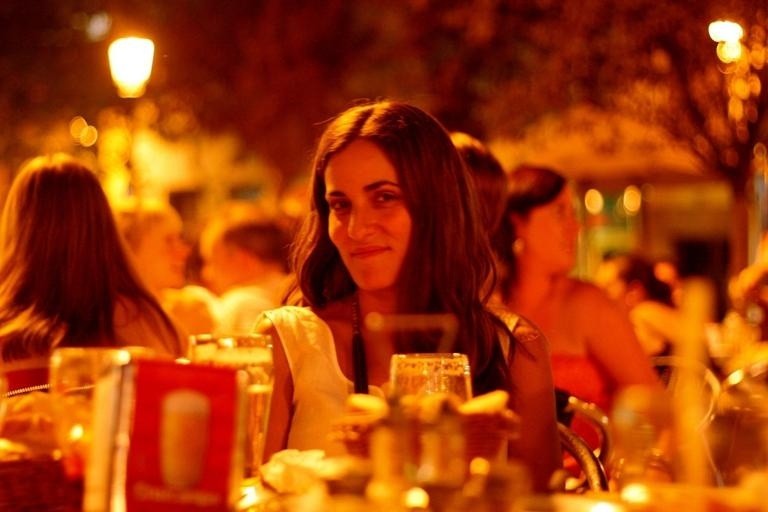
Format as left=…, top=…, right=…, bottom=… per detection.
left=555, top=422, right=609, bottom=497
left=555, top=390, right=612, bottom=464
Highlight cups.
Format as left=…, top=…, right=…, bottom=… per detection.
left=390, top=353, right=473, bottom=400
left=46, top=345, right=132, bottom=479
left=187, top=333, right=274, bottom=485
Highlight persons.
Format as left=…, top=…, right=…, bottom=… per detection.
left=263, top=101, right=565, bottom=490
left=122, top=202, right=290, bottom=293
left=453, top=131, right=659, bottom=439
left=0, top=156, right=180, bottom=357
